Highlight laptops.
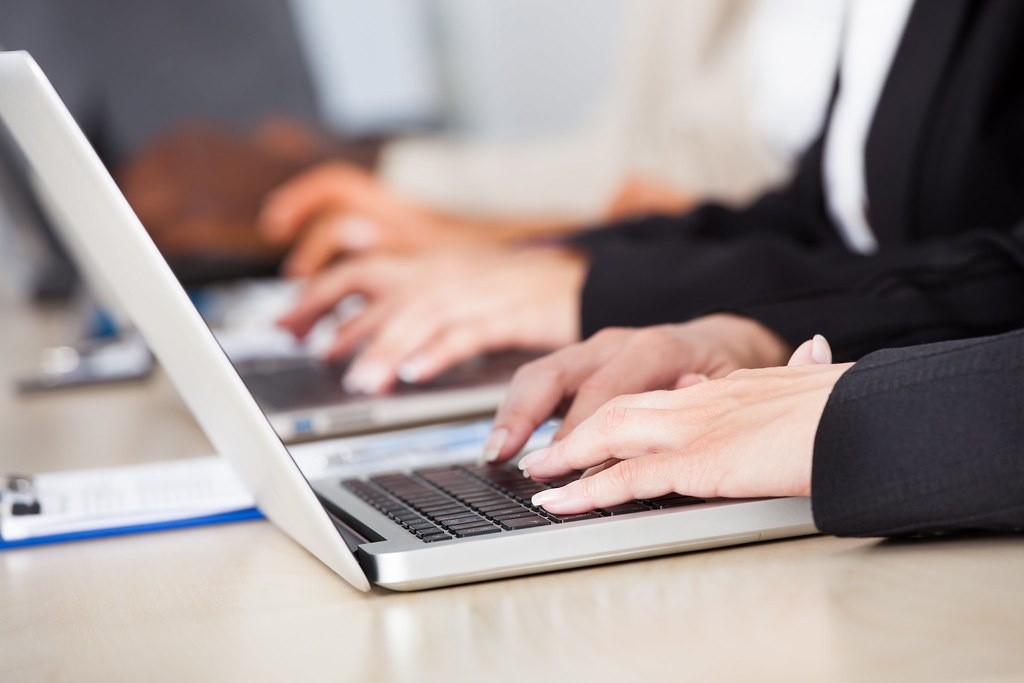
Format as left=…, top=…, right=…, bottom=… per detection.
left=0, top=50, right=822, bottom=594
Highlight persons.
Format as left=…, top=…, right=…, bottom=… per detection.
left=107, top=2, right=1024, bottom=537
